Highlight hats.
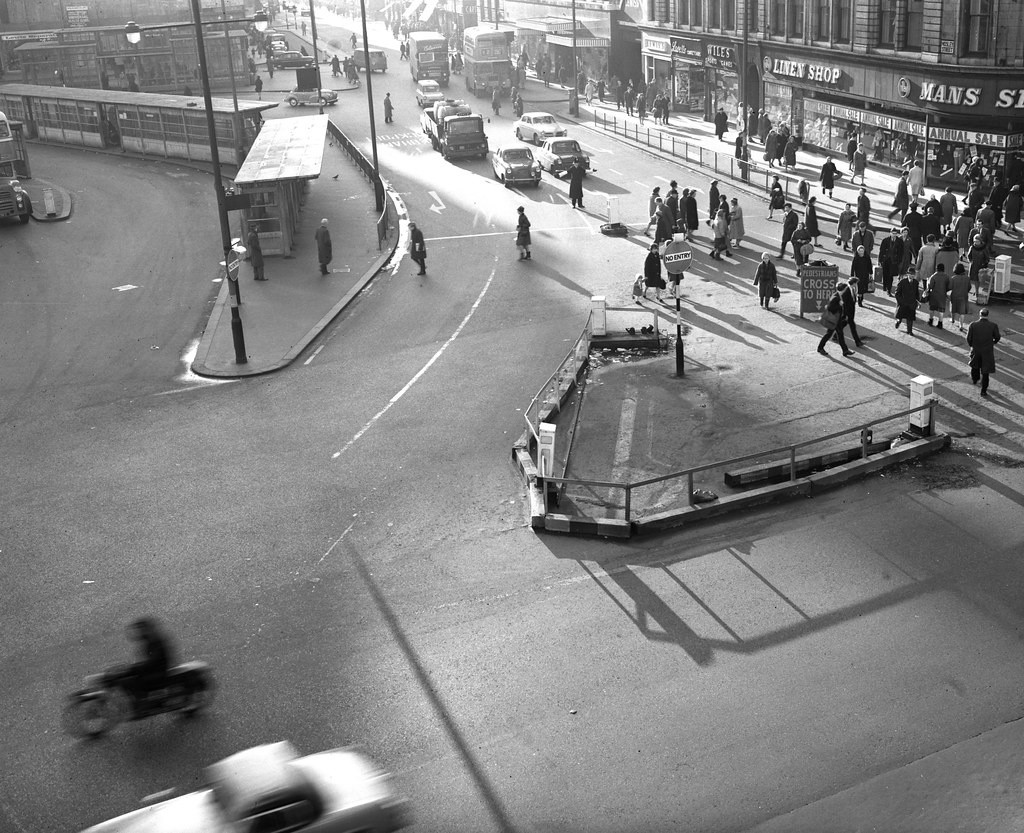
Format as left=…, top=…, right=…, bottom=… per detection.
left=945, top=230, right=955, bottom=237
left=993, top=177, right=1000, bottom=182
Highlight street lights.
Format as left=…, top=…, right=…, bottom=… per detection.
left=125, top=0, right=271, bottom=306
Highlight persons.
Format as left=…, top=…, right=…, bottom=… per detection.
left=255, top=76, right=263, bottom=101
left=184, top=84, right=192, bottom=96
left=384, top=92, right=394, bottom=123
left=315, top=218, right=333, bottom=275
left=301, top=22, right=307, bottom=36
left=554, top=157, right=596, bottom=209
left=247, top=26, right=274, bottom=79
left=285, top=41, right=288, bottom=51
left=247, top=224, right=269, bottom=282
left=98, top=71, right=139, bottom=92
left=408, top=223, right=428, bottom=275
left=515, top=206, right=532, bottom=261
left=384, top=15, right=530, bottom=88
left=113, top=614, right=175, bottom=704
left=610, top=75, right=1024, bottom=395
left=300, top=46, right=308, bottom=57
left=515, top=48, right=606, bottom=106
left=349, top=33, right=358, bottom=48
left=330, top=55, right=360, bottom=84
left=493, top=86, right=524, bottom=118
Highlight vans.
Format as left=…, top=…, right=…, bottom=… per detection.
left=355, top=47, right=388, bottom=72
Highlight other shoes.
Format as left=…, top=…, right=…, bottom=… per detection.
left=644, top=231, right=650, bottom=236
left=843, top=350, right=855, bottom=356
left=323, top=272, right=331, bottom=273
left=775, top=255, right=783, bottom=259
left=630, top=292, right=677, bottom=303
left=711, top=245, right=741, bottom=261
left=843, top=245, right=850, bottom=250
left=973, top=380, right=977, bottom=384
left=894, top=319, right=964, bottom=333
left=981, top=391, right=987, bottom=396
left=817, top=348, right=828, bottom=355
left=814, top=244, right=822, bottom=247
left=767, top=216, right=772, bottom=219
left=858, top=299, right=863, bottom=307
left=857, top=342, right=867, bottom=347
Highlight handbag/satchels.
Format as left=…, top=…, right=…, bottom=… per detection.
left=772, top=284, right=781, bottom=299
left=660, top=279, right=666, bottom=290
left=835, top=234, right=842, bottom=245
left=823, top=310, right=838, bottom=330
left=800, top=241, right=813, bottom=256
left=868, top=276, right=875, bottom=291
left=922, top=289, right=931, bottom=303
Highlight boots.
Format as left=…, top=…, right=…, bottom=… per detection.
left=519, top=252, right=531, bottom=260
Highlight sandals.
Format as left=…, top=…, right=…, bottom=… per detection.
left=418, top=272, right=426, bottom=275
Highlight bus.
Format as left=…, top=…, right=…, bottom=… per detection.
left=0, top=112, right=32, bottom=224
left=463, top=26, right=511, bottom=99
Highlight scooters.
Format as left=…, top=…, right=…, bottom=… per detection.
left=62, top=662, right=216, bottom=739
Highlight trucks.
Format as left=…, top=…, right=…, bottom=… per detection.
left=420, top=99, right=490, bottom=161
left=408, top=32, right=450, bottom=88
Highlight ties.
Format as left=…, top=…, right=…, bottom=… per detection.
left=892, top=237, right=894, bottom=245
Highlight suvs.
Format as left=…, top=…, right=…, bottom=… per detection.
left=416, top=79, right=444, bottom=108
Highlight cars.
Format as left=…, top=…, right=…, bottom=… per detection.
left=82, top=743, right=410, bottom=833
left=491, top=146, right=542, bottom=189
left=512, top=111, right=568, bottom=145
left=261, top=3, right=317, bottom=70
left=284, top=84, right=339, bottom=108
left=538, top=137, right=591, bottom=176
